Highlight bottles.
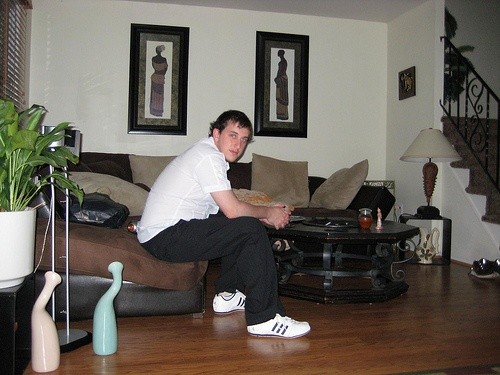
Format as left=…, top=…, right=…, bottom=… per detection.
left=358, top=208, right=373, bottom=230
left=127, top=223, right=137, bottom=233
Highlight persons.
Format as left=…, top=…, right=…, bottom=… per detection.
left=137, top=110, right=311, bottom=340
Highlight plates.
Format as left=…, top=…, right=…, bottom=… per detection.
left=291, top=216, right=306, bottom=221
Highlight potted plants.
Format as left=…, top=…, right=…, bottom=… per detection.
left=0, top=98, right=84, bottom=291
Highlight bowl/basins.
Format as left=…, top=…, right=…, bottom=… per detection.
left=473, top=258, right=496, bottom=274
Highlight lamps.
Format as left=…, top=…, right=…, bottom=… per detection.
left=399, top=128, right=462, bottom=218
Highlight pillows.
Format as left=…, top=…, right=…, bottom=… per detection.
left=56, top=152, right=368, bottom=216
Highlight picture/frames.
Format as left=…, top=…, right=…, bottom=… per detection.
left=398, top=66, right=416, bottom=101
left=127, top=23, right=190, bottom=136
left=254, top=31, right=310, bottom=138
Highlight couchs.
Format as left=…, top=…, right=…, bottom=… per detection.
left=30, top=151, right=395, bottom=319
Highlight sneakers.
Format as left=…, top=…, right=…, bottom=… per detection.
left=491, top=259, right=500, bottom=278
left=212, top=289, right=247, bottom=313
left=467, top=258, right=496, bottom=279
left=247, top=312, right=312, bottom=339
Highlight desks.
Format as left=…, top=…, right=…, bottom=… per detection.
left=265, top=220, right=421, bottom=304
left=398, top=214, right=452, bottom=265
left=0, top=275, right=40, bottom=375
